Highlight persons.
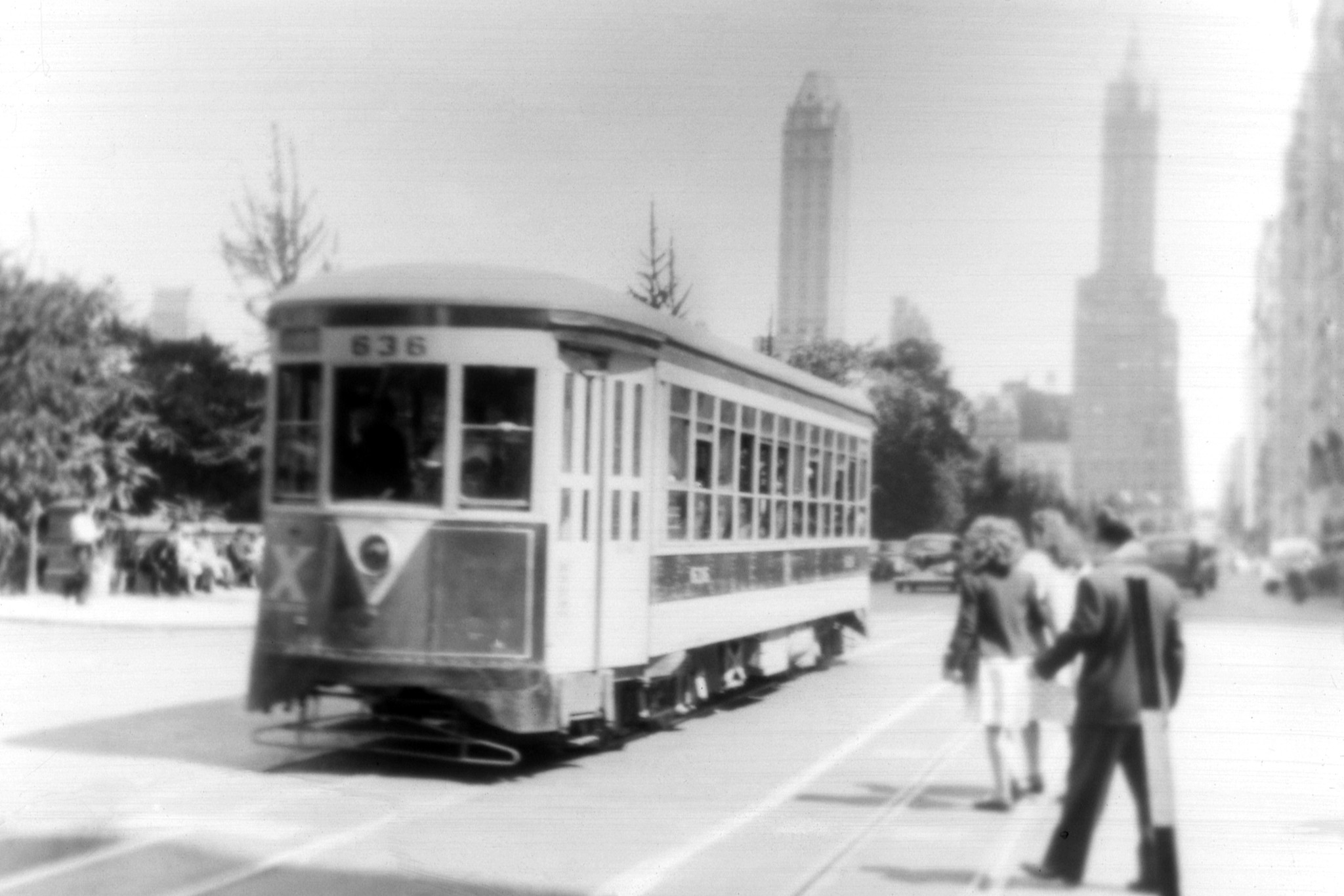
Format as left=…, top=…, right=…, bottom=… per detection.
left=143, top=520, right=236, bottom=594
left=232, top=525, right=267, bottom=588
left=943, top=514, right=1055, bottom=809
left=1021, top=497, right=1095, bottom=681
left=1030, top=502, right=1189, bottom=896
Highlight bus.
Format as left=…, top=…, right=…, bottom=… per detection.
left=245, top=267, right=879, bottom=771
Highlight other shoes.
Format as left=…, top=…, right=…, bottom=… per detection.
left=1129, top=877, right=1178, bottom=896
left=1022, top=860, right=1078, bottom=888
left=973, top=799, right=1011, bottom=812
left=1028, top=777, right=1042, bottom=793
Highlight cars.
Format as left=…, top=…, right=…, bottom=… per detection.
left=1145, top=533, right=1219, bottom=599
left=867, top=533, right=964, bottom=594
left=1216, top=538, right=1343, bottom=603
